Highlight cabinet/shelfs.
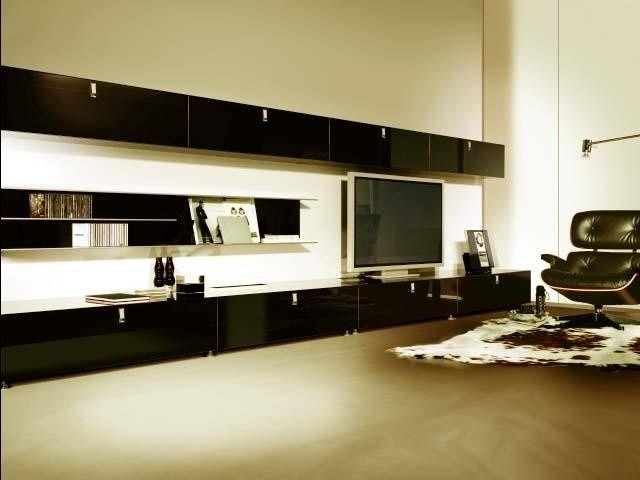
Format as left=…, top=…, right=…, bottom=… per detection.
left=1, top=268, right=531, bottom=388
left=0, top=189, right=321, bottom=250
left=0, top=65, right=504, bottom=180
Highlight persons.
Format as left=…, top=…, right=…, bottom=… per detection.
left=196, top=200, right=215, bottom=245
left=239, top=207, right=250, bottom=226
left=231, top=207, right=239, bottom=215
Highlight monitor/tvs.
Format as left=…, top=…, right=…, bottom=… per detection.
left=346, top=171, right=445, bottom=279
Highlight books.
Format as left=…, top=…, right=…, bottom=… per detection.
left=28, top=193, right=96, bottom=220
left=86, top=289, right=170, bottom=306
left=71, top=222, right=129, bottom=247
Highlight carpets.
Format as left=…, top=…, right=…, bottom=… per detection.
left=390, top=307, right=639, bottom=373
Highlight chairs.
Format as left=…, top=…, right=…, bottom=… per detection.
left=541, top=210, right=639, bottom=329
